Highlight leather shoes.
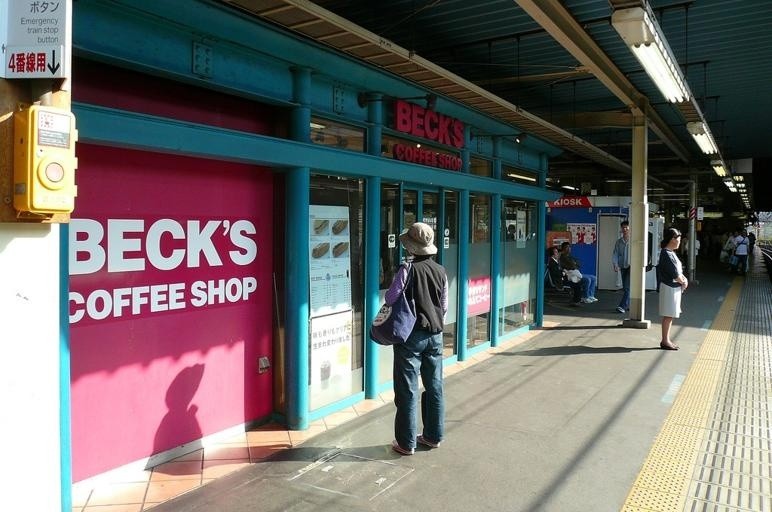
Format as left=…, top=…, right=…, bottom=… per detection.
left=660, top=340, right=681, bottom=350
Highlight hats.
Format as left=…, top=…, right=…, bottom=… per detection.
left=400, top=222, right=438, bottom=256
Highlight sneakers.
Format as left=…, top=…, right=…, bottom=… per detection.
left=417, top=434, right=440, bottom=447
left=615, top=306, right=626, bottom=314
left=581, top=296, right=598, bottom=304
left=392, top=440, right=414, bottom=455
left=574, top=302, right=584, bottom=307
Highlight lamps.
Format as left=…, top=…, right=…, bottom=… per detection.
left=608, top=1, right=754, bottom=211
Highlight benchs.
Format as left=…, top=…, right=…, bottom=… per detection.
left=547, top=242, right=600, bottom=307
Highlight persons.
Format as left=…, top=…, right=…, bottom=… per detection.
left=612, top=220, right=630, bottom=315
left=590, top=227, right=597, bottom=243
left=658, top=228, right=688, bottom=350
left=384, top=222, right=448, bottom=455
left=576, top=226, right=586, bottom=244
left=506, top=224, right=516, bottom=241
left=546, top=242, right=598, bottom=307
left=720, top=228, right=756, bottom=275
left=685, top=240, right=700, bottom=268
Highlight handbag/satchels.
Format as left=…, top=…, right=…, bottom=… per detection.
left=368, top=290, right=418, bottom=346
left=729, top=254, right=739, bottom=265
